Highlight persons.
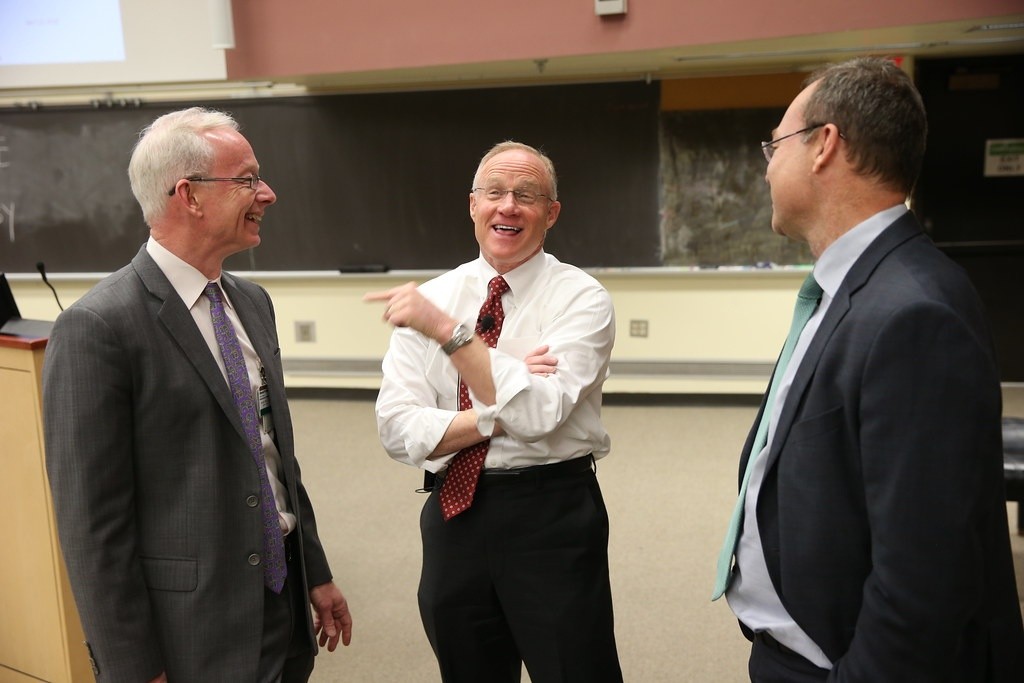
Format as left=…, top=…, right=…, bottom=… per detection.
left=42, top=106, right=353, bottom=683
left=711, top=57, right=1024, bottom=683
left=362, top=140, right=623, bottom=682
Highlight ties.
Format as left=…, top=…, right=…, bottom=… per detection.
left=440, top=277, right=512, bottom=521
left=202, top=282, right=287, bottom=595
left=712, top=272, right=824, bottom=599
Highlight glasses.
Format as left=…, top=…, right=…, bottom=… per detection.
left=472, top=186, right=555, bottom=207
left=169, top=173, right=260, bottom=194
left=762, top=123, right=849, bottom=162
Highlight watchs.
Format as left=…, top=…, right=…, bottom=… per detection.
left=440, top=322, right=473, bottom=355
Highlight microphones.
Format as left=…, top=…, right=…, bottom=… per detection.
left=37, top=261, right=63, bottom=311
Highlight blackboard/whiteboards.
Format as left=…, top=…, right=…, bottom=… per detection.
left=0, top=15, right=1024, bottom=282
left=337, top=264, right=386, bottom=273
